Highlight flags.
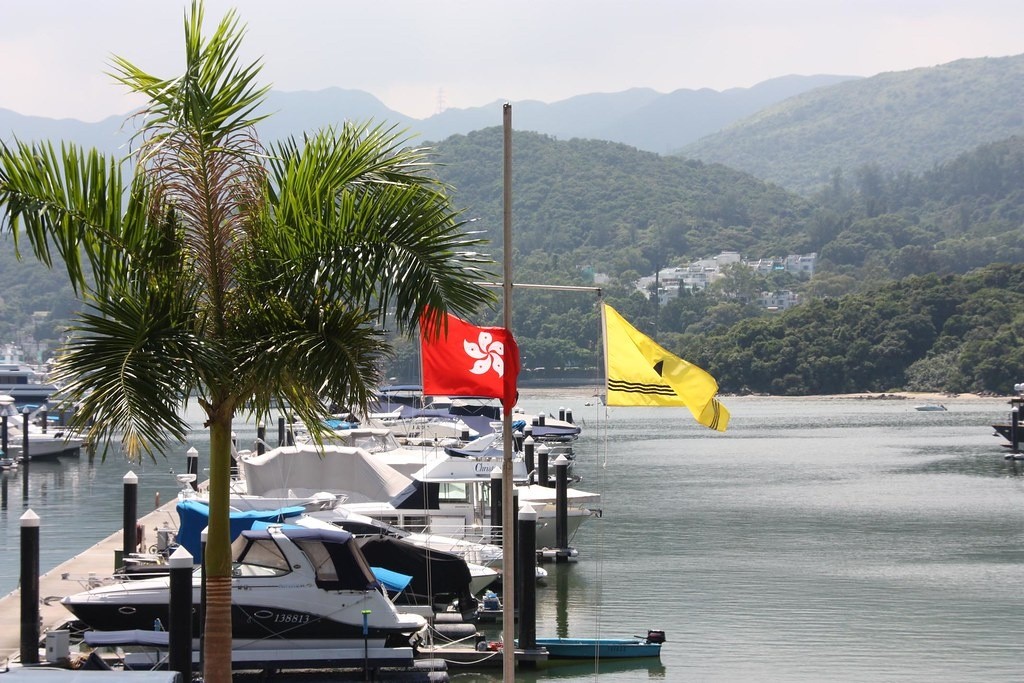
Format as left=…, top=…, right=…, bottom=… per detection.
left=418, top=305, right=520, bottom=417
left=601, top=303, right=730, bottom=432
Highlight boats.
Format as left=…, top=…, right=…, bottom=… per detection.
left=176, top=384, right=603, bottom=612
left=0, top=364, right=60, bottom=402
left=8, top=435, right=83, bottom=457
left=992, top=421, right=1024, bottom=442
left=915, top=404, right=948, bottom=411
left=61, top=525, right=427, bottom=670
